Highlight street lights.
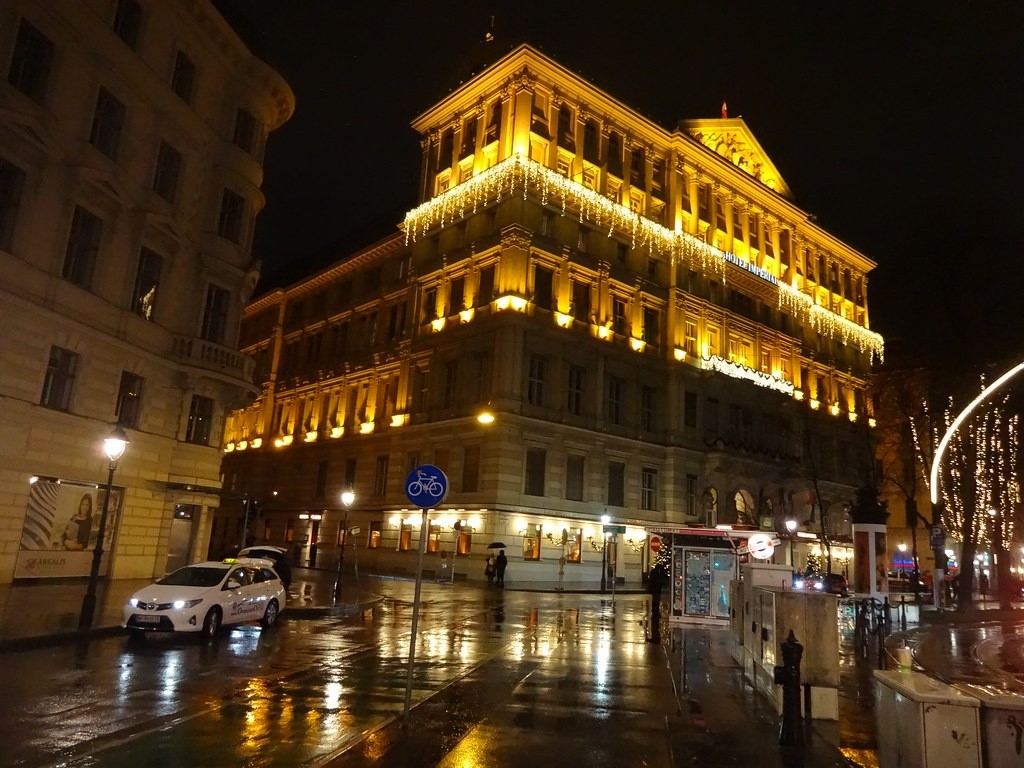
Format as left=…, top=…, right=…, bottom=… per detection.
left=333, top=482, right=356, bottom=607
left=976, top=552, right=983, bottom=575
left=784, top=516, right=797, bottom=586
left=78, top=422, right=131, bottom=646
left=601, top=508, right=609, bottom=592
left=898, top=540, right=906, bottom=588
left=241, top=487, right=277, bottom=550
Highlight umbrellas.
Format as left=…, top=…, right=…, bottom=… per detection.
left=487, top=542, right=506, bottom=552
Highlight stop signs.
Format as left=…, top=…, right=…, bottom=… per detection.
left=650, top=537, right=660, bottom=551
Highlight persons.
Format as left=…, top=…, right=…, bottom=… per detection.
left=909, top=570, right=916, bottom=592
left=495, top=550, right=507, bottom=588
left=485, top=553, right=497, bottom=586
left=650, top=563, right=665, bottom=614
left=63, top=494, right=92, bottom=550
left=951, top=575, right=959, bottom=599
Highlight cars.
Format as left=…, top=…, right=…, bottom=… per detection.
left=797, top=572, right=849, bottom=597
left=1017, top=580, right=1024, bottom=597
left=888, top=568, right=918, bottom=578
left=121, top=546, right=289, bottom=640
left=923, top=566, right=958, bottom=586
